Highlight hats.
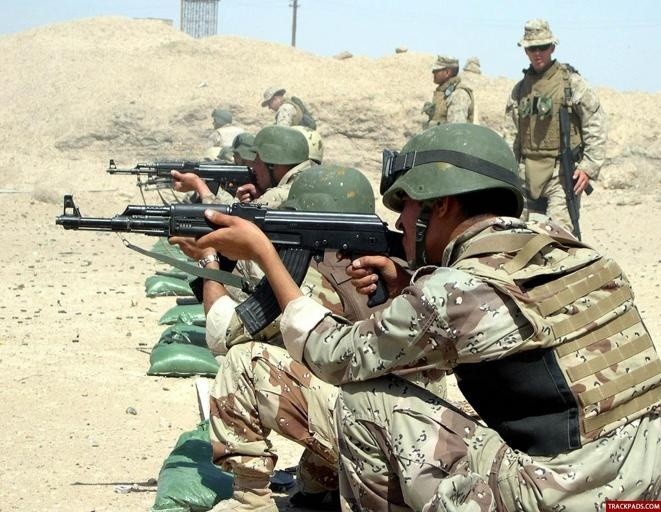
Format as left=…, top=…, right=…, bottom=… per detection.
left=433, top=55, right=459, bottom=70
left=261, top=87, right=286, bottom=107
left=517, top=19, right=554, bottom=47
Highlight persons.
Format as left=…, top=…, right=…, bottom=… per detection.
left=262, top=86, right=316, bottom=130
left=184, top=125, right=661, bottom=510
left=501, top=19, right=608, bottom=242
left=422, top=55, right=474, bottom=130
left=174, top=107, right=447, bottom=512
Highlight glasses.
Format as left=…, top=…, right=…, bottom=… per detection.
left=528, top=44, right=550, bottom=52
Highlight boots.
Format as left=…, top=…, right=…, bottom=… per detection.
left=205, top=475, right=279, bottom=512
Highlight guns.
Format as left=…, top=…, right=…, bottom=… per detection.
left=555, top=105, right=594, bottom=242
left=106, top=159, right=266, bottom=202
left=55, top=195, right=428, bottom=335
